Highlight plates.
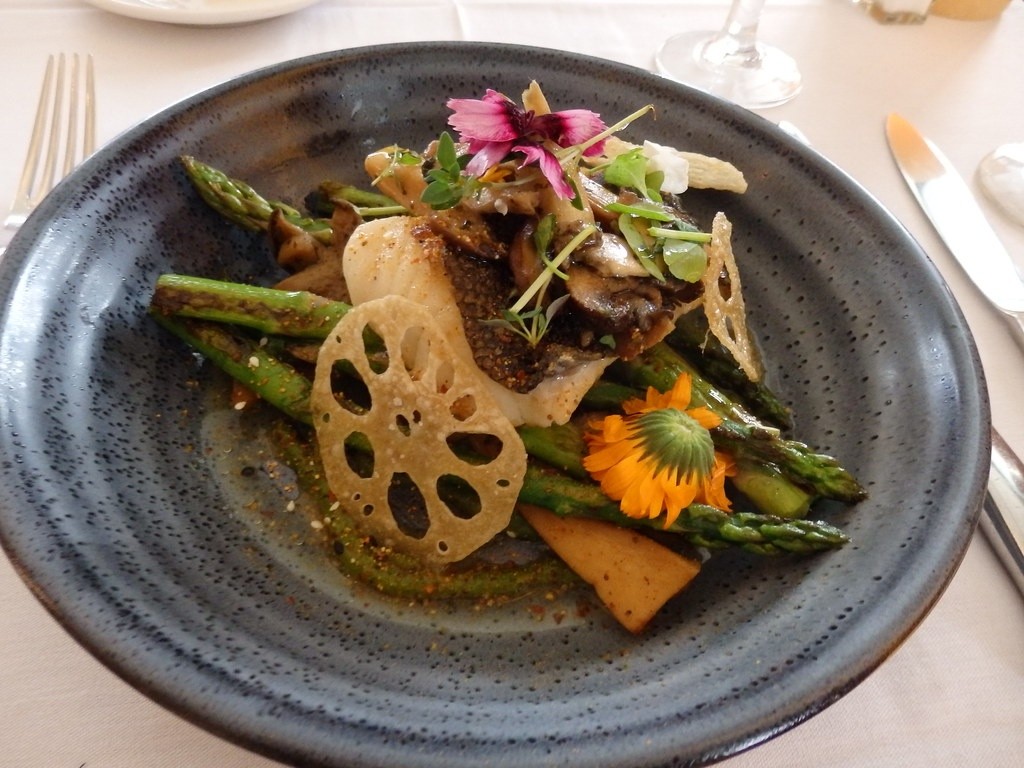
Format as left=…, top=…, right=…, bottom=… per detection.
left=0, top=38, right=993, bottom=767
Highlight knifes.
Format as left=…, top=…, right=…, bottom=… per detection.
left=886, top=109, right=1019, bottom=355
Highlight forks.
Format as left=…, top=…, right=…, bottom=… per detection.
left=0, top=51, right=97, bottom=261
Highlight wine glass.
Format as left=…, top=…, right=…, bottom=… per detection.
left=657, top=0, right=802, bottom=109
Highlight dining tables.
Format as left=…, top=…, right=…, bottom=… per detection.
left=0, top=0, right=1024, bottom=768
